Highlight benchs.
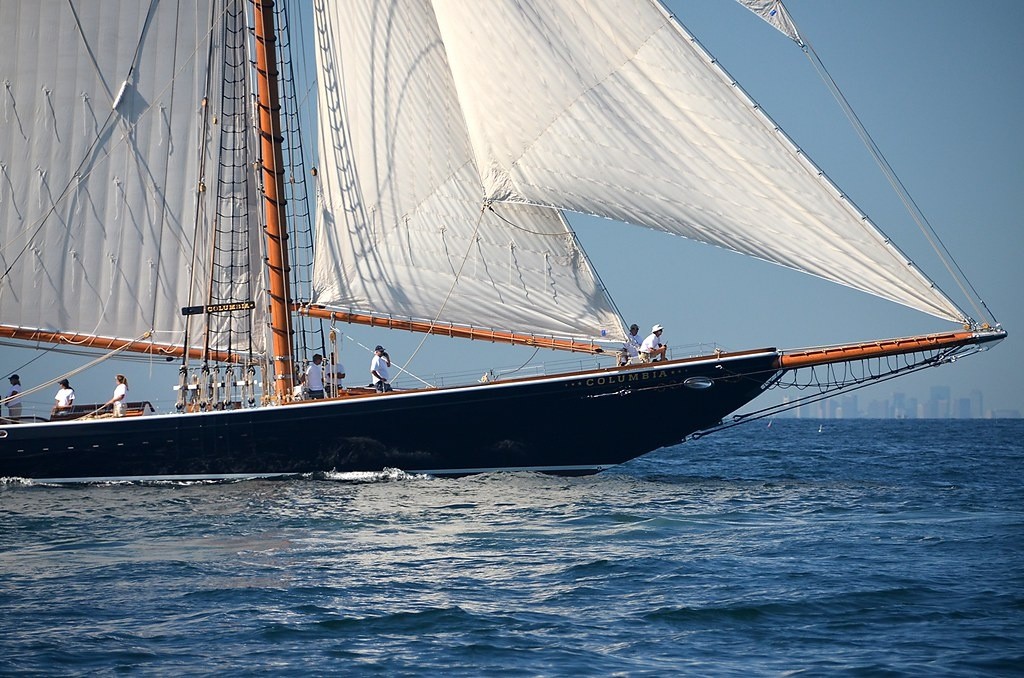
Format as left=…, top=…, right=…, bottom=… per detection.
left=50, top=401, right=155, bottom=421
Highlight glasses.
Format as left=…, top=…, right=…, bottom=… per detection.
left=60, top=383, right=62, bottom=385
left=658, top=329, right=662, bottom=332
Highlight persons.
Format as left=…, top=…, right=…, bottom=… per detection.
left=306, top=354, right=324, bottom=399
left=325, top=353, right=345, bottom=394
left=105, top=374, right=129, bottom=417
left=621, top=324, right=643, bottom=366
left=640, top=324, right=667, bottom=362
left=55, top=379, right=75, bottom=412
left=371, top=345, right=393, bottom=392
left=5, top=374, right=22, bottom=415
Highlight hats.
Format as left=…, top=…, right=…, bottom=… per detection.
left=631, top=324, right=639, bottom=328
left=652, top=324, right=663, bottom=333
left=375, top=346, right=386, bottom=352
left=58, top=379, right=69, bottom=384
left=8, top=374, right=19, bottom=379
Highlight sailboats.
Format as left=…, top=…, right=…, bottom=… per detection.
left=0, top=0, right=1010, bottom=484
